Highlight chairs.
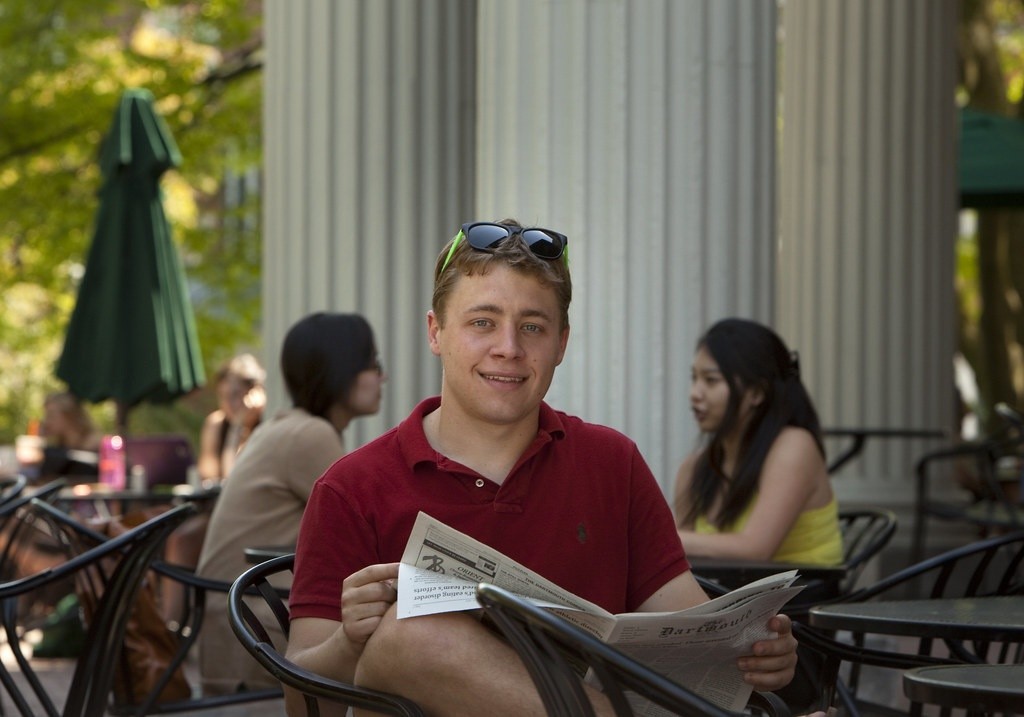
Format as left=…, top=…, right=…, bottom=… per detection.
left=0, top=427, right=1024, bottom=717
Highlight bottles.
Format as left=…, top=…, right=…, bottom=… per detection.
left=132, top=464, right=146, bottom=495
left=99, top=435, right=126, bottom=492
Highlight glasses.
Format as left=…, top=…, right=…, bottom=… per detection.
left=441, top=222, right=568, bottom=275
left=365, top=361, right=381, bottom=377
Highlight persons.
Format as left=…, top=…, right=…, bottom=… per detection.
left=200, top=314, right=387, bottom=694
left=0, top=350, right=268, bottom=634
left=671, top=317, right=843, bottom=599
left=283, top=219, right=799, bottom=717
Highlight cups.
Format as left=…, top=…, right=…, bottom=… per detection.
left=15, top=435, right=42, bottom=481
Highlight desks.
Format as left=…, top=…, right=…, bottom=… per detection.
left=901, top=662, right=1024, bottom=717
left=808, top=597, right=1023, bottom=670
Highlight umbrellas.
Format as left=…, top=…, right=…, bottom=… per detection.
left=54, top=87, right=206, bottom=440
